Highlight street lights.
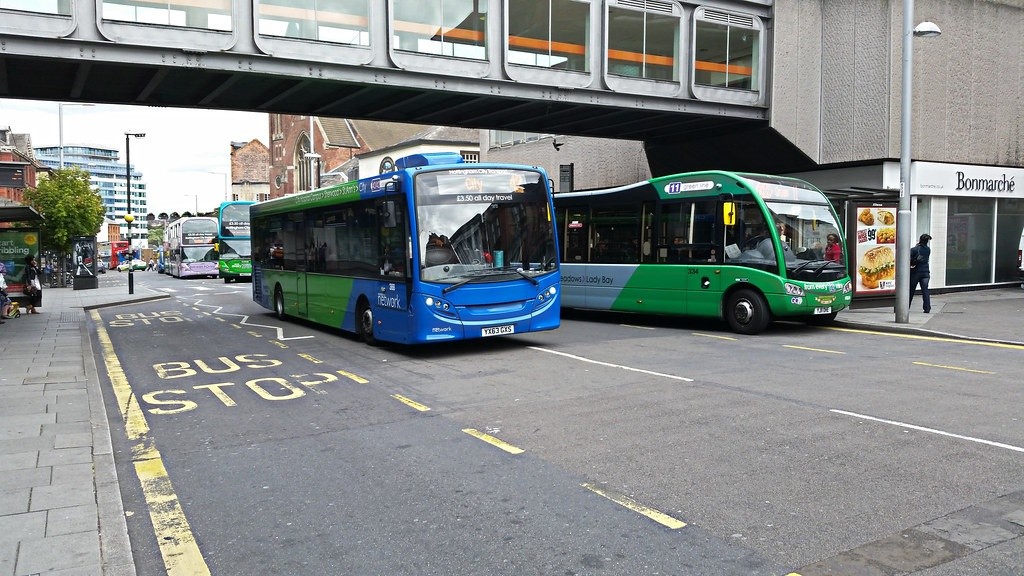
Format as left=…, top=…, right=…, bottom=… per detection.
left=895, top=21, right=942, bottom=323
left=59, top=104, right=96, bottom=288
left=124, top=214, right=135, bottom=295
left=126, top=133, right=147, bottom=292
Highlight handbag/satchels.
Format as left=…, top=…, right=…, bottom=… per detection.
left=23, top=285, right=37, bottom=297
left=910, top=244, right=925, bottom=268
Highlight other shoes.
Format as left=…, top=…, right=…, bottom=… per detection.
left=31, top=309, right=40, bottom=314
left=924, top=309, right=930, bottom=313
left=25, top=305, right=31, bottom=315
left=0, top=322, right=5, bottom=324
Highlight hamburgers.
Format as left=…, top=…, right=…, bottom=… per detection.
left=859, top=246, right=894, bottom=288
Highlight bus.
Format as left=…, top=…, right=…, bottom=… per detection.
left=215, top=201, right=255, bottom=283
left=250, top=152, right=559, bottom=345
left=167, top=216, right=219, bottom=279
left=156, top=230, right=169, bottom=273
left=550, top=170, right=852, bottom=334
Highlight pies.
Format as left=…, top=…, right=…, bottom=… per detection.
left=877, top=210, right=895, bottom=225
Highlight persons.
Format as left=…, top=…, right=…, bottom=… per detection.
left=147, top=258, right=154, bottom=271
left=24, top=254, right=42, bottom=314
left=0, top=263, right=8, bottom=324
left=909, top=234, right=932, bottom=314
left=672, top=236, right=685, bottom=243
left=825, top=234, right=841, bottom=263
left=427, top=233, right=443, bottom=247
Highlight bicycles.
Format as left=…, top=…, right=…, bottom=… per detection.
left=41, top=267, right=74, bottom=287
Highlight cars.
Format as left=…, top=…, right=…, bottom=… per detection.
left=117, top=258, right=146, bottom=271
left=98, top=259, right=106, bottom=273
